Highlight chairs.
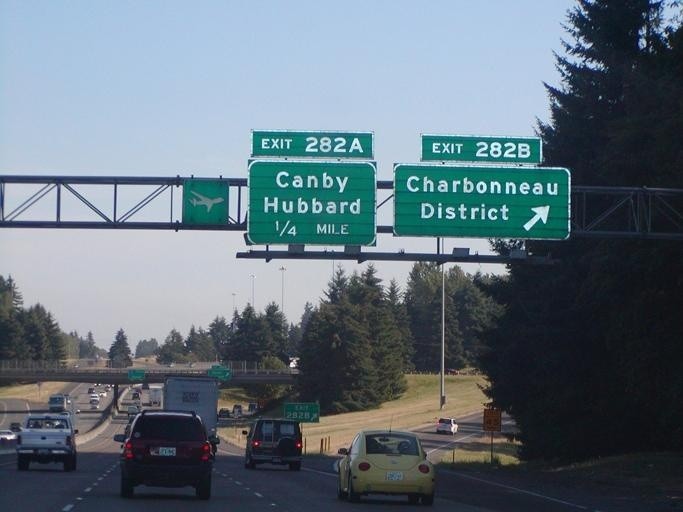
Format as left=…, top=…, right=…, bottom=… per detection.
left=367, top=438, right=412, bottom=451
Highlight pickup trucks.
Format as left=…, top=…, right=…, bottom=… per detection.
left=15, top=414, right=78, bottom=470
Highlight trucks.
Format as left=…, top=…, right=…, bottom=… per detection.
left=162, top=374, right=219, bottom=457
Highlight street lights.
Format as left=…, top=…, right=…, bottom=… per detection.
left=280, top=265, right=286, bottom=314
left=233, top=292, right=236, bottom=314
left=249, top=274, right=256, bottom=309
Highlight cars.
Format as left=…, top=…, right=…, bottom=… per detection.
left=336, top=427, right=435, bottom=504
left=127, top=383, right=149, bottom=416
left=0, top=422, right=22, bottom=443
left=220, top=403, right=242, bottom=417
left=88, top=383, right=114, bottom=409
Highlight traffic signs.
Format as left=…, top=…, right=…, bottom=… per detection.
left=250, top=159, right=376, bottom=246
left=127, top=369, right=145, bottom=381
left=284, top=403, right=319, bottom=422
left=394, top=164, right=570, bottom=242
left=208, top=368, right=231, bottom=380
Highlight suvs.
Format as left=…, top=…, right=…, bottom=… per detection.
left=242, top=417, right=303, bottom=470
left=437, top=418, right=458, bottom=435
left=114, top=409, right=212, bottom=499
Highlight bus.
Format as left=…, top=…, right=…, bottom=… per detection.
left=49, top=394, right=66, bottom=412
